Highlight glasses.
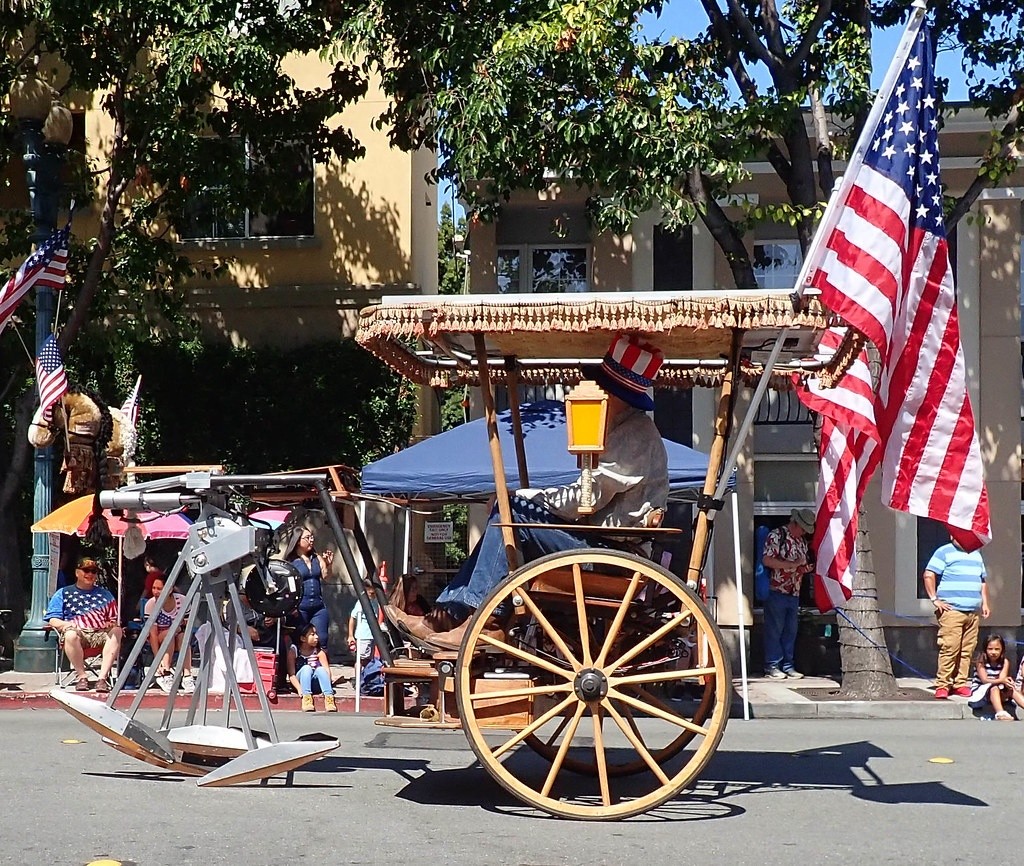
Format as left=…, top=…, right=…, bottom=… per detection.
left=79, top=568, right=96, bottom=574
left=302, top=535, right=314, bottom=541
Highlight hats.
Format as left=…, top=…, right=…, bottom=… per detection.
left=791, top=509, right=816, bottom=534
left=577, top=334, right=666, bottom=412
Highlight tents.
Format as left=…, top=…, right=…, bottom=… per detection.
left=360, top=400, right=749, bottom=723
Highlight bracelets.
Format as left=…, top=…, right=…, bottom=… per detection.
left=931, top=596, right=938, bottom=602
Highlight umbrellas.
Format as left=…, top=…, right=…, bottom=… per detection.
left=235, top=510, right=291, bottom=530
left=30, top=493, right=195, bottom=681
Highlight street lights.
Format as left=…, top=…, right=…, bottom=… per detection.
left=9, top=75, right=74, bottom=673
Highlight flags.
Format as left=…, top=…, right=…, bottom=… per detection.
left=788, top=311, right=881, bottom=614
left=0, top=206, right=71, bottom=333
left=36, top=332, right=70, bottom=423
left=120, top=383, right=139, bottom=429
left=793, top=8, right=992, bottom=553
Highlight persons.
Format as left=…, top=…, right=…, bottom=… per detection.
left=763, top=509, right=815, bottom=680
left=136, top=557, right=163, bottom=623
left=379, top=334, right=669, bottom=654
left=923, top=534, right=990, bottom=699
left=227, top=525, right=337, bottom=711
left=967, top=635, right=1024, bottom=721
left=387, top=574, right=432, bottom=616
left=43, top=557, right=122, bottom=693
left=1015, top=656, right=1024, bottom=696
left=349, top=579, right=384, bottom=675
left=144, top=574, right=195, bottom=693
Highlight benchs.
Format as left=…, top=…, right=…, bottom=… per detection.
left=496, top=496, right=694, bottom=654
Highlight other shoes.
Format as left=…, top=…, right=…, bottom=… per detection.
left=95, top=679, right=110, bottom=692
left=935, top=689, right=948, bottom=698
left=995, top=711, right=1015, bottom=721
left=75, top=678, right=90, bottom=691
left=360, top=689, right=370, bottom=696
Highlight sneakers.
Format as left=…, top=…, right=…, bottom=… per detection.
left=954, top=686, right=972, bottom=697
left=787, top=670, right=804, bottom=679
left=324, top=696, right=337, bottom=712
left=302, top=695, right=314, bottom=711
left=156, top=675, right=174, bottom=693
left=182, top=676, right=196, bottom=692
left=764, top=667, right=787, bottom=679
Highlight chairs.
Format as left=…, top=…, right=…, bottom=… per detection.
left=40, top=621, right=118, bottom=692
left=130, top=597, right=199, bottom=688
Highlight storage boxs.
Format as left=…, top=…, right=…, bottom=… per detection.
left=238, top=651, right=280, bottom=693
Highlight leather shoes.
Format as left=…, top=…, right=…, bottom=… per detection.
left=384, top=605, right=454, bottom=654
left=425, top=614, right=508, bottom=652
